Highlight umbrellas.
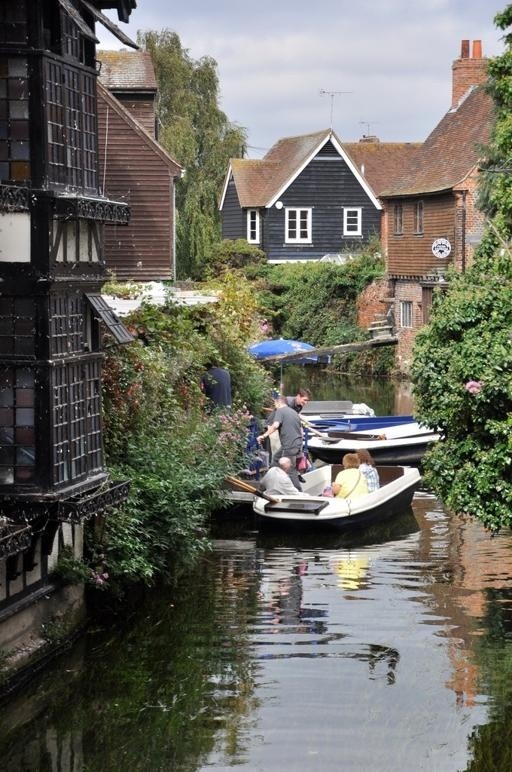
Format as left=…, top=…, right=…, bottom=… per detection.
left=247, top=337, right=331, bottom=395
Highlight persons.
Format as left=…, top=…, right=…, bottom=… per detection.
left=256, top=387, right=312, bottom=495
left=322, top=449, right=380, bottom=498
left=202, top=358, right=232, bottom=414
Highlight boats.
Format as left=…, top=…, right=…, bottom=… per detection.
left=252, top=462, right=421, bottom=531
left=279, top=397, right=447, bottom=464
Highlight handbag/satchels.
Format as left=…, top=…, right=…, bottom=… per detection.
left=295, top=455, right=312, bottom=473
